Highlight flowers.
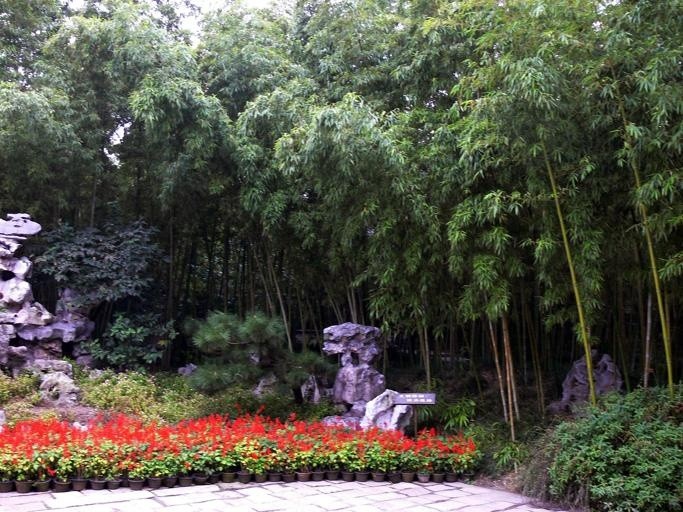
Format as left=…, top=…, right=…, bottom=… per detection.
left=0, top=403, right=484, bottom=478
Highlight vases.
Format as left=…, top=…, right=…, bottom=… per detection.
left=148, top=468, right=461, bottom=486
left=15, top=480, right=33, bottom=494
left=71, top=480, right=85, bottom=490
left=33, top=478, right=52, bottom=491
left=53, top=479, right=72, bottom=492
left=127, top=479, right=146, bottom=490
left=108, top=478, right=123, bottom=490
left=89, top=479, right=107, bottom=490
left=1, top=480, right=14, bottom=493
left=0, top=468, right=471, bottom=494
